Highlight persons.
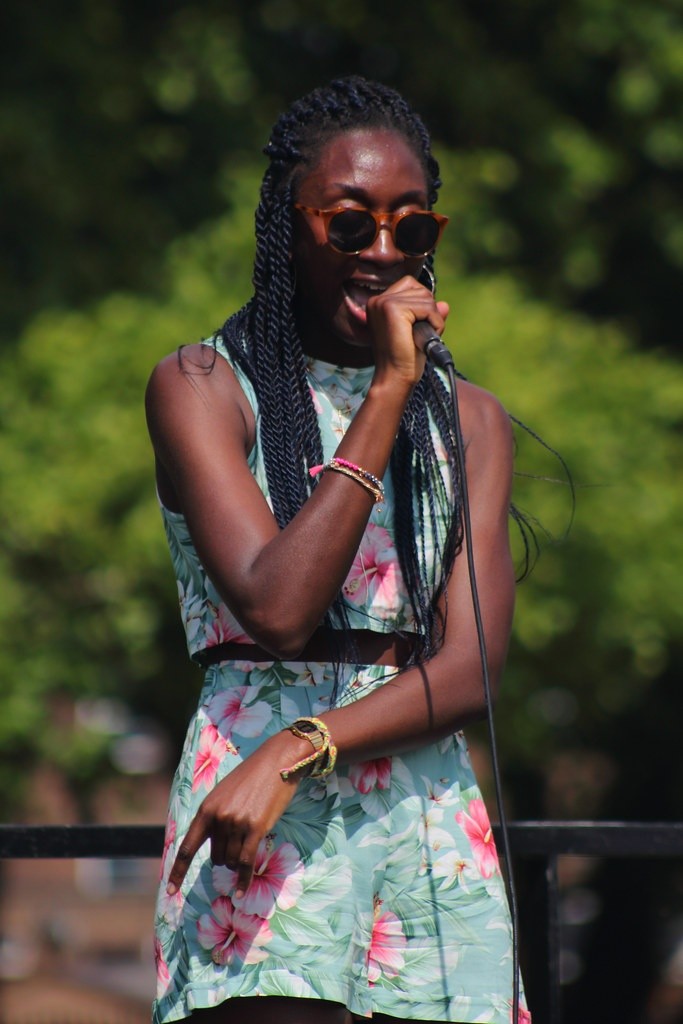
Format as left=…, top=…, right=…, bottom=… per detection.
left=0, top=685, right=683, bottom=1024
left=144, top=74, right=531, bottom=1024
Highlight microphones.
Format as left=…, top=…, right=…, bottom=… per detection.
left=413, top=320, right=455, bottom=373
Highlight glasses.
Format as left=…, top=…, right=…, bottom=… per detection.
left=294, top=201, right=449, bottom=259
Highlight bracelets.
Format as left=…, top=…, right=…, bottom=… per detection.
left=280, top=717, right=337, bottom=779
left=310, top=458, right=384, bottom=502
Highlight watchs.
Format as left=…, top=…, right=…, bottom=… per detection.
left=283, top=721, right=324, bottom=775
